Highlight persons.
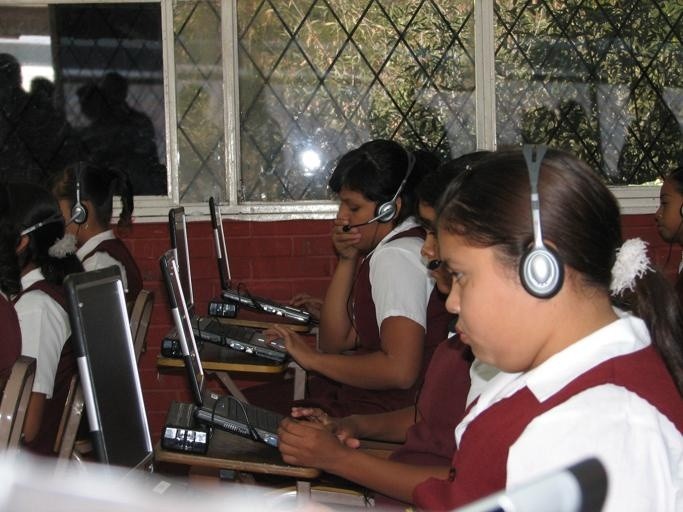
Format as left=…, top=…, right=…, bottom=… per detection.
left=0, top=52, right=168, bottom=460
left=240, top=139, right=455, bottom=421
left=412, top=143, right=683, bottom=511
left=174, top=77, right=683, bottom=208
left=654, top=164, right=683, bottom=302
left=275, top=151, right=494, bottom=512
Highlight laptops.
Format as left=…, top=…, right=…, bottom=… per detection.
left=64, top=265, right=214, bottom=510
left=206, top=196, right=313, bottom=322
left=167, top=205, right=288, bottom=363
left=158, top=249, right=301, bottom=449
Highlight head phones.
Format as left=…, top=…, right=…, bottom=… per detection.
left=375, top=147, right=417, bottom=223
left=66, top=161, right=93, bottom=225
left=8, top=213, right=62, bottom=252
left=521, top=142, right=565, bottom=300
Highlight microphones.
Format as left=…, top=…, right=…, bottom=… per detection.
left=208, top=283, right=264, bottom=318
left=67, top=212, right=80, bottom=228
left=343, top=214, right=386, bottom=236
left=161, top=395, right=258, bottom=456
left=161, top=310, right=225, bottom=359
left=426, top=260, right=443, bottom=271
